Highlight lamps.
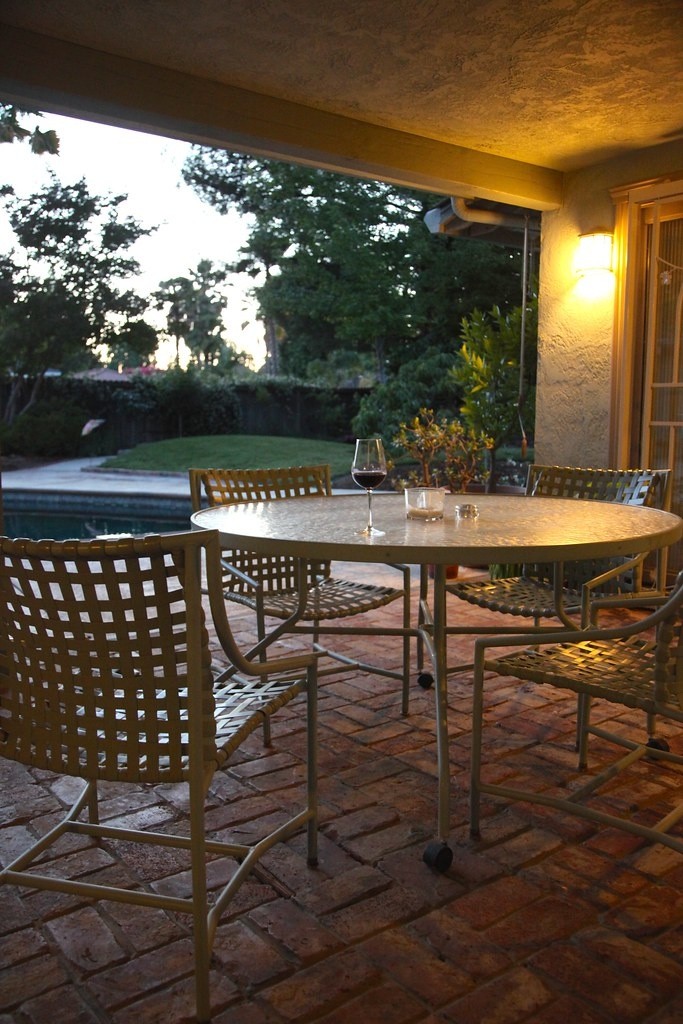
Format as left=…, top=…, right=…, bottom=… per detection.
left=576, top=228, right=615, bottom=273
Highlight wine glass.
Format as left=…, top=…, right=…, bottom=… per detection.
left=350, top=439, right=388, bottom=538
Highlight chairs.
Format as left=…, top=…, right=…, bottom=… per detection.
left=185, top=464, right=412, bottom=749
left=1, top=528, right=320, bottom=1024
left=469, top=575, right=683, bottom=855
left=444, top=463, right=675, bottom=750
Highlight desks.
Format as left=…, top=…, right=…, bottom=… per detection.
left=190, top=492, right=683, bottom=874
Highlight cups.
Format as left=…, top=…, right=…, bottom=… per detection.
left=404, top=487, right=445, bottom=521
